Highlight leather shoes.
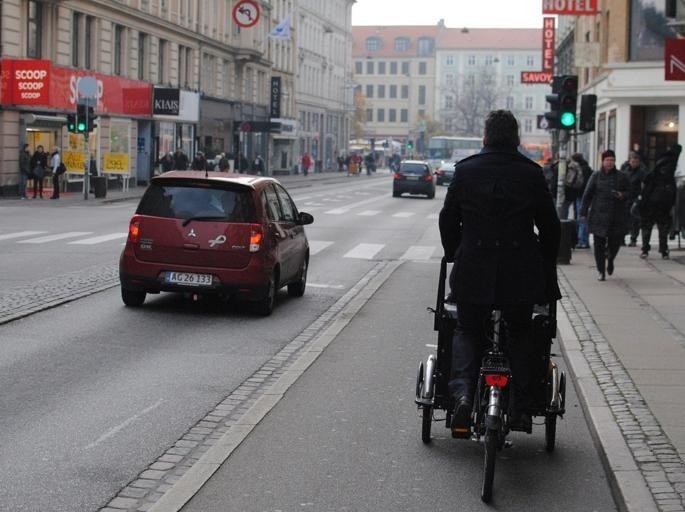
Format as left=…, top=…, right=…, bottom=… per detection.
left=509, top=413, right=532, bottom=432
left=598, top=260, right=614, bottom=280
left=451, top=397, right=472, bottom=432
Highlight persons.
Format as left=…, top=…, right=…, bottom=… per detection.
left=303, top=153, right=310, bottom=176
left=19, top=144, right=31, bottom=199
left=158, top=147, right=265, bottom=174
left=438, top=109, right=562, bottom=439
left=50, top=146, right=61, bottom=200
left=337, top=153, right=401, bottom=177
left=561, top=150, right=676, bottom=283
left=31, top=145, right=47, bottom=198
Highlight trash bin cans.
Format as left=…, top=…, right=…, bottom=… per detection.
left=556, top=220, right=573, bottom=265
left=94, top=176, right=107, bottom=198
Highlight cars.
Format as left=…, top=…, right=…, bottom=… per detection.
left=435, top=162, right=458, bottom=186
left=119, top=146, right=314, bottom=316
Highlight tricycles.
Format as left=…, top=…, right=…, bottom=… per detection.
left=415, top=252, right=568, bottom=504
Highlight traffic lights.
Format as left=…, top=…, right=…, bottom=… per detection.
left=545, top=76, right=559, bottom=130
left=88, top=106, right=97, bottom=132
left=67, top=114, right=75, bottom=133
left=580, top=92, right=599, bottom=133
left=559, top=75, right=578, bottom=131
left=407, top=140, right=413, bottom=150
left=77, top=104, right=85, bottom=133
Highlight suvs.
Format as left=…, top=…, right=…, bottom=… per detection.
left=393, top=159, right=437, bottom=199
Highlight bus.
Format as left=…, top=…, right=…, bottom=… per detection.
left=424, top=136, right=485, bottom=171
left=517, top=144, right=553, bottom=170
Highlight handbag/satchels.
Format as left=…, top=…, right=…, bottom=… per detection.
left=33, top=165, right=45, bottom=178
left=51, top=163, right=66, bottom=175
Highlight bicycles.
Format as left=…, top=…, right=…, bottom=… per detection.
left=672, top=175, right=685, bottom=251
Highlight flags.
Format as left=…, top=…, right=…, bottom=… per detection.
left=271, top=16, right=292, bottom=40
